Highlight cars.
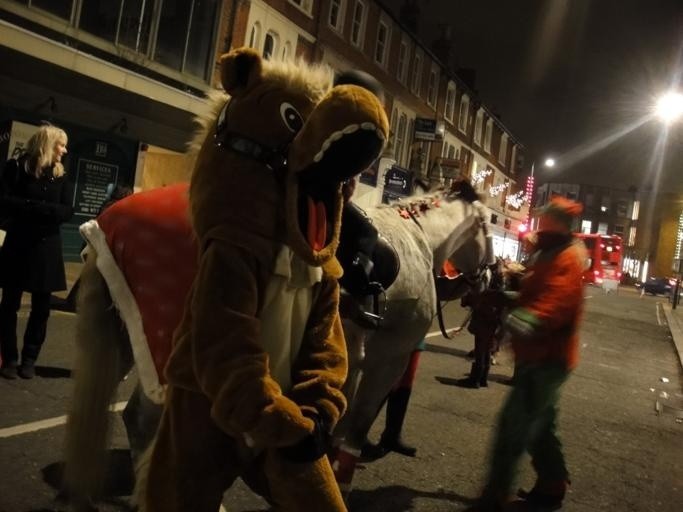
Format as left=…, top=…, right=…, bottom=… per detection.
left=638, top=277, right=677, bottom=295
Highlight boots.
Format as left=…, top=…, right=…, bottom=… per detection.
left=518, top=479, right=569, bottom=509
left=459, top=361, right=491, bottom=388
left=362, top=388, right=416, bottom=455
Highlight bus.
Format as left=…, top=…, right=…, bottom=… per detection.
left=573, top=232, right=623, bottom=285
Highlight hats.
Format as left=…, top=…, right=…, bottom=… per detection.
left=537, top=196, right=580, bottom=224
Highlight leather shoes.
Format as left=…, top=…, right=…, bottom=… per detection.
left=2, top=361, right=34, bottom=379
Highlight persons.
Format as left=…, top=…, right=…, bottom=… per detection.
left=455, top=290, right=505, bottom=390
left=63, top=182, right=135, bottom=311
left=465, top=195, right=589, bottom=511
left=0, top=123, right=74, bottom=380
left=342, top=266, right=489, bottom=460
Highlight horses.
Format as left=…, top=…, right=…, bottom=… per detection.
left=61, top=174, right=494, bottom=500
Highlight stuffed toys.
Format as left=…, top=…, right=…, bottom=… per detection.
left=136, top=45, right=392, bottom=510
